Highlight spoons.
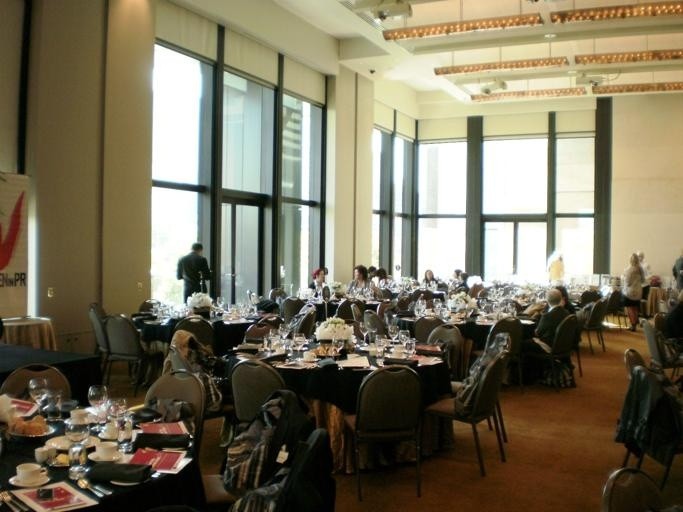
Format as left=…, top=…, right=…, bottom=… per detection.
left=0, top=489, right=31, bottom=511
left=75, top=473, right=114, bottom=499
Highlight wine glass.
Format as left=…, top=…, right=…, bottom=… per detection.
left=28, top=377, right=134, bottom=486
left=147, top=294, right=259, bottom=323
left=293, top=276, right=412, bottom=303
left=415, top=276, right=599, bottom=323
left=263, top=316, right=417, bottom=367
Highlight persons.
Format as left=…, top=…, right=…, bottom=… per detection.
left=309, top=265, right=469, bottom=311
left=177, top=243, right=213, bottom=305
left=516, top=252, right=683, bottom=383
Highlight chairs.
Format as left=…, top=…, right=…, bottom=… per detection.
left=654, top=311, right=676, bottom=359
left=604, top=467, right=668, bottom=512
left=633, top=366, right=683, bottom=490
left=642, top=321, right=683, bottom=381
left=624, top=347, right=665, bottom=468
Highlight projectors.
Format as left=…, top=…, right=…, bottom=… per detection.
left=577, top=76, right=603, bottom=86
left=478, top=79, right=506, bottom=97
left=373, top=4, right=413, bottom=21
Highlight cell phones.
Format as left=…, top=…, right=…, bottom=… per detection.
left=38, top=488, right=53, bottom=501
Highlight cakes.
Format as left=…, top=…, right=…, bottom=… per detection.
left=315, top=317, right=352, bottom=340
left=187, top=291, right=212, bottom=308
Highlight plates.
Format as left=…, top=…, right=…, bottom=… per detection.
left=5, top=410, right=194, bottom=489
